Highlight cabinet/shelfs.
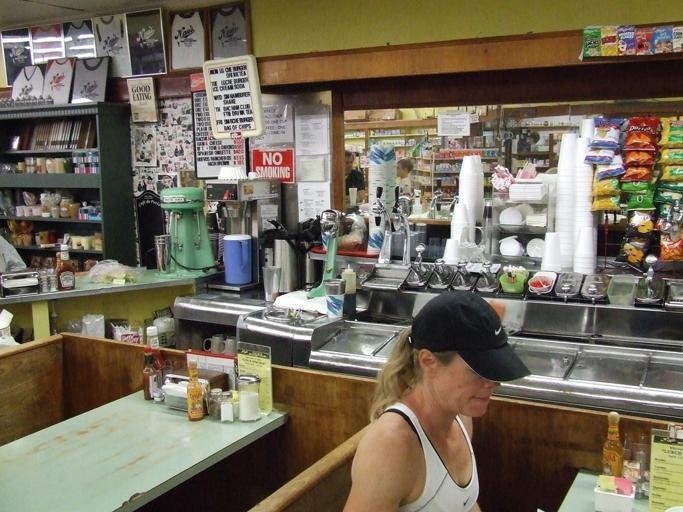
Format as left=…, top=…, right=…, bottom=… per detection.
left=187, top=360, right=205, bottom=419
left=141, top=324, right=162, bottom=402
left=342, top=264, right=359, bottom=317
left=209, top=388, right=234, bottom=423
left=57, top=243, right=74, bottom=290
left=236, top=376, right=264, bottom=422
left=12, top=150, right=98, bottom=176
left=601, top=411, right=623, bottom=478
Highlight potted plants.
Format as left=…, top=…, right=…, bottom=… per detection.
left=0, top=1, right=252, bottom=93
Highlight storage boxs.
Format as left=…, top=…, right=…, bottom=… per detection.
left=246, top=415, right=378, bottom=512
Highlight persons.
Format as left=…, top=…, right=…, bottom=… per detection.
left=342, top=292, right=533, bottom=512
left=392, top=159, right=414, bottom=216
left=344, top=150, right=368, bottom=204
left=0, top=235, right=28, bottom=273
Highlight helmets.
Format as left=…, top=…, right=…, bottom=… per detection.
left=497, top=204, right=545, bottom=258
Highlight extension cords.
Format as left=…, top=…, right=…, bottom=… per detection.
left=221, top=234, right=252, bottom=284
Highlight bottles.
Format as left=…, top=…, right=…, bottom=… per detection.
left=411, top=290, right=533, bottom=383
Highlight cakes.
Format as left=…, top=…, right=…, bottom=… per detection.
left=39, top=231, right=57, bottom=243
left=59, top=195, right=74, bottom=218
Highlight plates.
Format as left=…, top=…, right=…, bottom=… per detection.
left=342, top=104, right=588, bottom=203
left=1, top=100, right=138, bottom=268
left=490, top=174, right=558, bottom=269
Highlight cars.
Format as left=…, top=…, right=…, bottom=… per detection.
left=443, top=155, right=485, bottom=264
left=410, top=223, right=453, bottom=262
left=203, top=335, right=239, bottom=359
left=349, top=187, right=358, bottom=206
left=261, top=265, right=282, bottom=305
left=154, top=235, right=171, bottom=274
left=541, top=117, right=598, bottom=275
left=324, top=278, right=344, bottom=321
